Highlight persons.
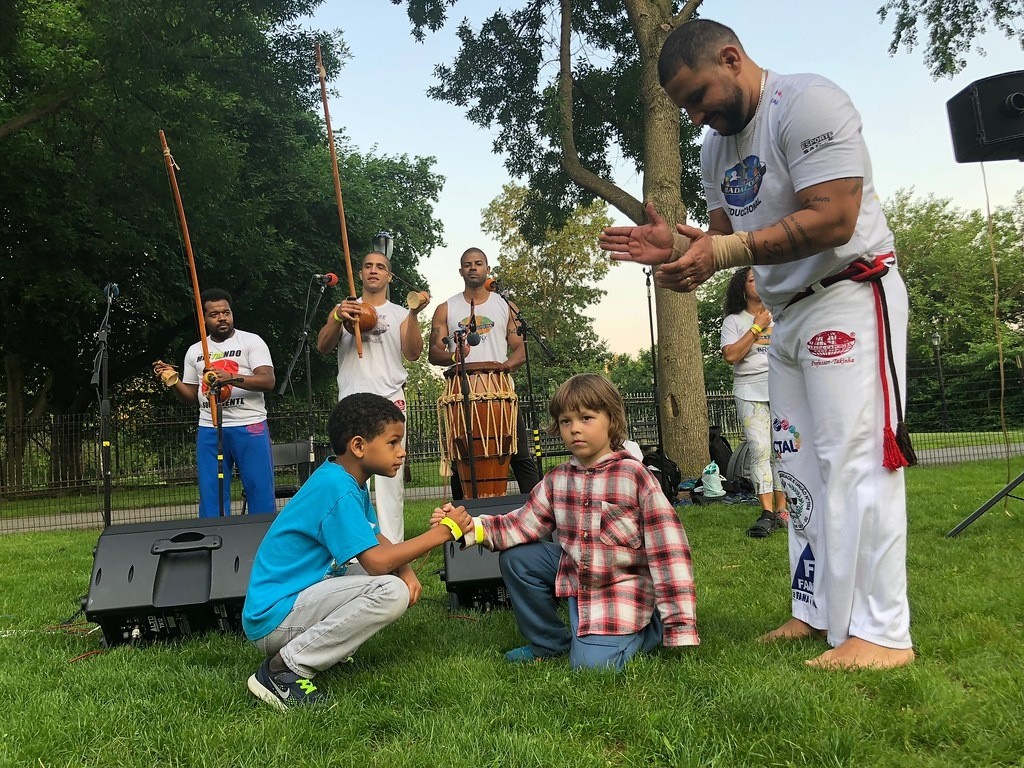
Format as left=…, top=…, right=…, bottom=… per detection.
left=242, top=393, right=473, bottom=711
left=596, top=18, right=917, bottom=670
left=428, top=247, right=539, bottom=499
left=430, top=374, right=701, bottom=672
left=319, top=251, right=431, bottom=543
left=152, top=289, right=278, bottom=517
left=721, top=267, right=788, bottom=528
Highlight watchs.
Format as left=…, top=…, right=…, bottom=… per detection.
left=750, top=327, right=758, bottom=337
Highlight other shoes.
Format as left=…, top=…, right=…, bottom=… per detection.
left=756, top=510, right=776, bottom=527
left=505, top=645, right=537, bottom=663
left=775, top=508, right=789, bottom=527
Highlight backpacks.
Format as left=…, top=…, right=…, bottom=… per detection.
left=692, top=425, right=752, bottom=505
left=642, top=450, right=681, bottom=505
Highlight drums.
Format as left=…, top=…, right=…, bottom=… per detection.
left=443, top=361, right=517, bottom=500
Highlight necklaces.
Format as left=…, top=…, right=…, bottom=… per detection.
left=736, top=68, right=765, bottom=168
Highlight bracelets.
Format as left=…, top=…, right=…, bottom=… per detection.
left=452, top=354, right=456, bottom=362
left=712, top=231, right=754, bottom=270
left=334, top=312, right=343, bottom=322
left=472, top=517, right=483, bottom=543
left=669, top=233, right=690, bottom=264
left=230, top=373, right=233, bottom=378
left=752, top=324, right=762, bottom=332
left=440, top=518, right=463, bottom=541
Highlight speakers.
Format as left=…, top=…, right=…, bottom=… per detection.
left=439, top=493, right=559, bottom=612
left=86, top=512, right=280, bottom=648
left=947, top=69, right=1024, bottom=164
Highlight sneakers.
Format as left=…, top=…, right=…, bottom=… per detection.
left=337, top=657, right=364, bottom=673
left=248, top=657, right=324, bottom=713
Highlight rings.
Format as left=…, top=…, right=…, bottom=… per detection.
left=686, top=277, right=691, bottom=286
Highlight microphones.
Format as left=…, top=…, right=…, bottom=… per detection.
left=314, top=273, right=338, bottom=286
left=104, top=283, right=120, bottom=297
left=484, top=278, right=501, bottom=292
left=467, top=298, right=480, bottom=346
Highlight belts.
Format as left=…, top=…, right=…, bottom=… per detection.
left=783, top=260, right=896, bottom=309
